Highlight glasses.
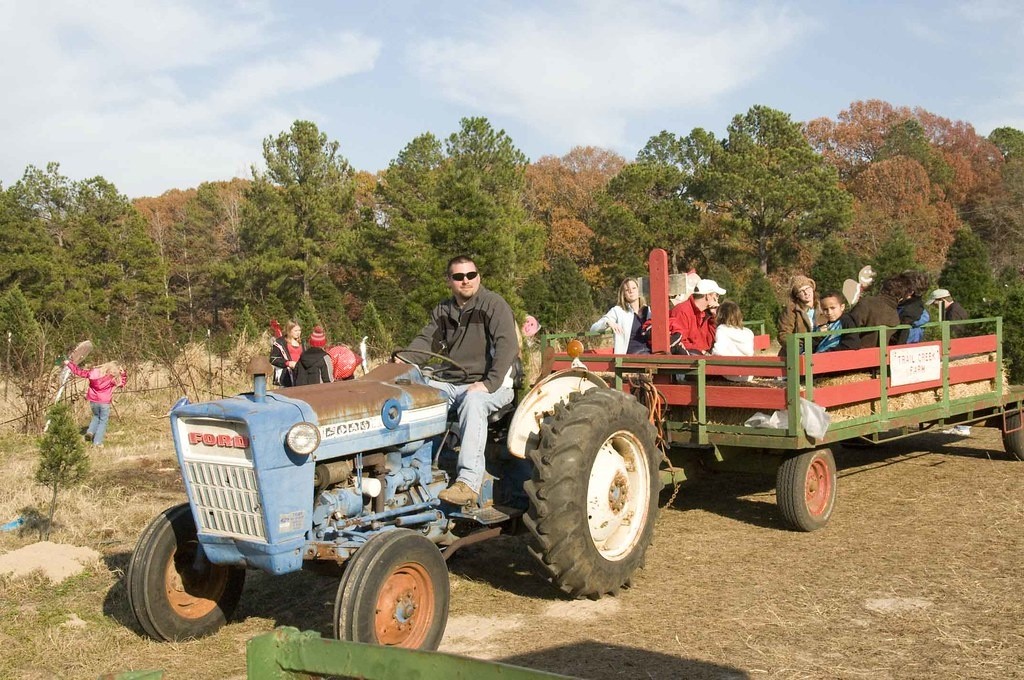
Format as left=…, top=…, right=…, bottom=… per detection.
left=797, top=286, right=812, bottom=295
left=449, top=272, right=478, bottom=280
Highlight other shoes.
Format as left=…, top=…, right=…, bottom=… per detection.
left=98, top=444, right=104, bottom=447
left=84, top=433, right=93, bottom=441
left=943, top=426, right=970, bottom=435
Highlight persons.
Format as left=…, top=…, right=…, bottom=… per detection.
left=670, top=279, right=726, bottom=361
left=270, top=319, right=303, bottom=388
left=292, top=326, right=334, bottom=387
left=64, top=360, right=127, bottom=448
left=323, top=342, right=354, bottom=381
left=703, top=301, right=755, bottom=383
left=590, top=277, right=700, bottom=421
left=812, top=289, right=861, bottom=353
left=387, top=256, right=522, bottom=508
left=889, top=271, right=930, bottom=346
left=777, top=276, right=829, bottom=358
left=850, top=278, right=907, bottom=349
left=925, top=289, right=972, bottom=436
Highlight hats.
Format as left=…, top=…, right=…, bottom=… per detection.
left=925, top=289, right=950, bottom=306
left=693, top=279, right=726, bottom=295
left=789, top=275, right=816, bottom=300
left=310, top=326, right=326, bottom=346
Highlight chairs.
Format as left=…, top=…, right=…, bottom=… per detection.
left=448, top=357, right=521, bottom=422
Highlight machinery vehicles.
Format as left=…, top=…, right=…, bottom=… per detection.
left=125, top=249, right=1024, bottom=654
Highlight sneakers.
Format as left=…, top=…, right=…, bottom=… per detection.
left=438, top=481, right=478, bottom=507
